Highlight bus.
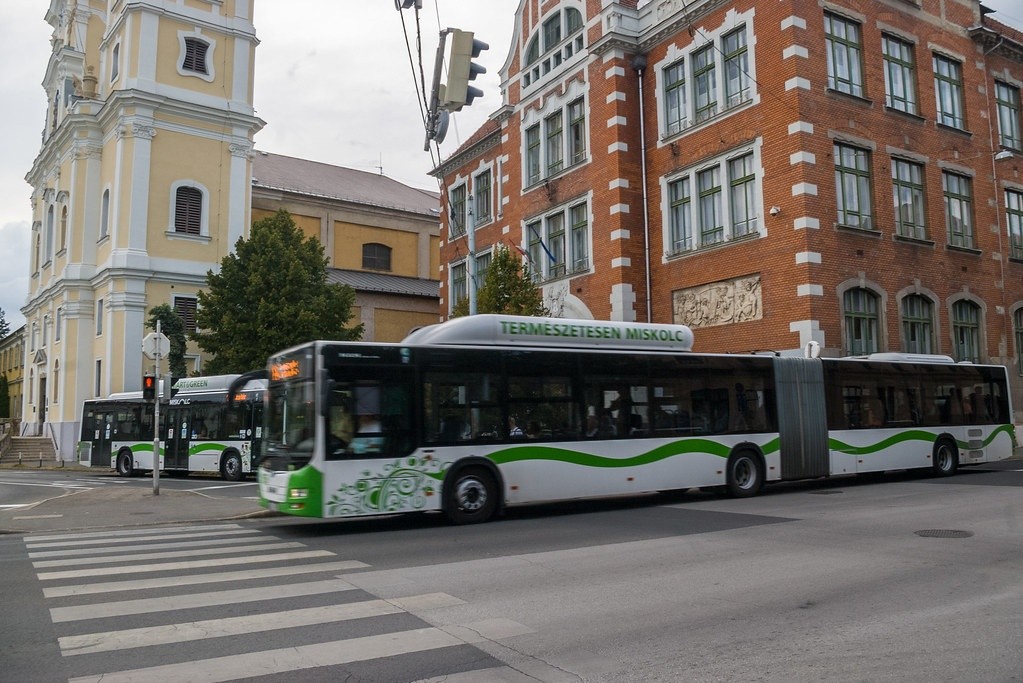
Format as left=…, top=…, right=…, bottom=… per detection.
left=224, top=313, right=1017, bottom=527
left=75, top=372, right=316, bottom=482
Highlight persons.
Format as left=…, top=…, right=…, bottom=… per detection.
left=735, top=382, right=748, bottom=423
left=460, top=381, right=632, bottom=440
left=859, top=386, right=1000, bottom=427
left=346, top=414, right=383, bottom=453
left=198, top=427, right=211, bottom=439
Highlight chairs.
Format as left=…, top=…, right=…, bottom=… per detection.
left=536, top=408, right=708, bottom=440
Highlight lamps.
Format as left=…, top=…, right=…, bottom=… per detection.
left=994, top=148, right=1016, bottom=162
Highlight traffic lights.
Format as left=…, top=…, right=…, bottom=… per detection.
left=143, top=374, right=156, bottom=400
left=438, top=29, right=490, bottom=114
left=162, top=374, right=180, bottom=402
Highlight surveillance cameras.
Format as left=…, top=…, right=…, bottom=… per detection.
left=769, top=209, right=777, bottom=216
